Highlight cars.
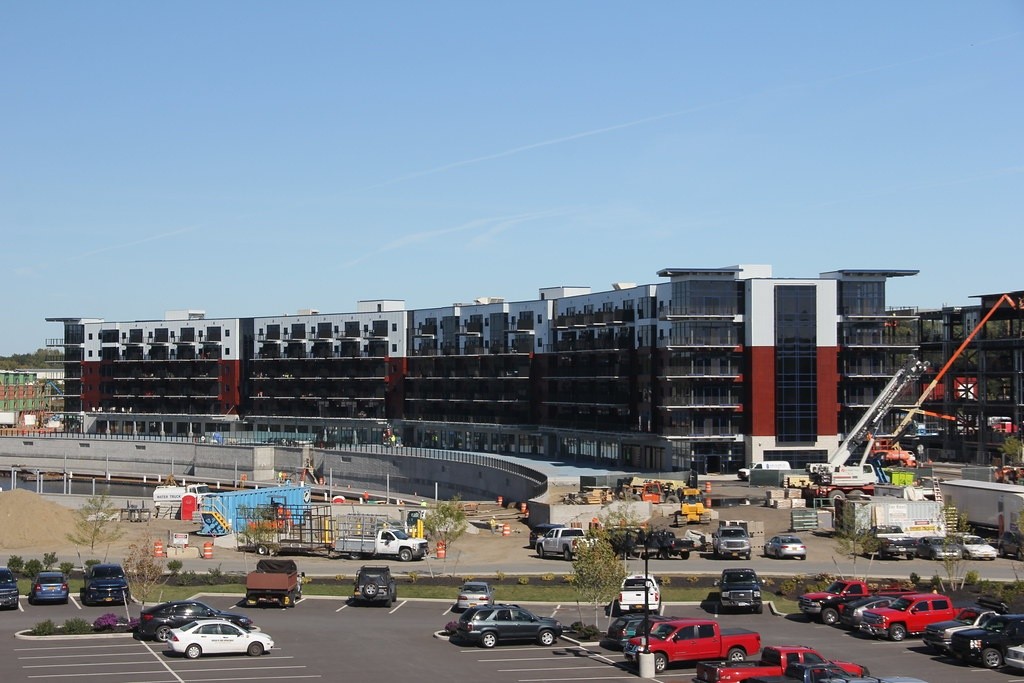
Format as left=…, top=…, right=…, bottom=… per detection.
left=839, top=594, right=908, bottom=630
left=138, top=599, right=254, bottom=642
left=0, top=567, right=20, bottom=610
left=166, top=615, right=275, bottom=660
left=457, top=581, right=495, bottom=613
left=917, top=535, right=963, bottom=561
left=31, top=571, right=69, bottom=605
left=1004, top=642, right=1024, bottom=669
left=867, top=525, right=917, bottom=560
left=764, top=535, right=807, bottom=560
left=954, top=535, right=997, bottom=561
left=998, top=530, right=1024, bottom=561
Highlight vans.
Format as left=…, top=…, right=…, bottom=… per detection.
left=738, top=460, right=791, bottom=481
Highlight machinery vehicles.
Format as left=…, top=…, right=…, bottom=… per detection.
left=677, top=488, right=711, bottom=527
left=627, top=481, right=681, bottom=504
left=247, top=495, right=294, bottom=533
left=805, top=293, right=1024, bottom=506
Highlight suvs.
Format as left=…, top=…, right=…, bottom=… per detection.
left=713, top=568, right=764, bottom=614
left=456, top=603, right=565, bottom=648
left=353, top=564, right=397, bottom=607
left=86, top=563, right=130, bottom=606
left=713, top=524, right=752, bottom=559
left=529, top=524, right=566, bottom=549
left=606, top=613, right=667, bottom=653
left=951, top=614, right=1024, bottom=669
left=636, top=616, right=680, bottom=637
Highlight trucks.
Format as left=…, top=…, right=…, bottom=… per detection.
left=152, top=483, right=210, bottom=508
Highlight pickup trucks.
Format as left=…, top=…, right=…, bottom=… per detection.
left=797, top=579, right=920, bottom=625
left=331, top=528, right=430, bottom=563
left=647, top=531, right=695, bottom=559
left=748, top=662, right=855, bottom=683
left=245, top=559, right=305, bottom=607
left=623, top=618, right=761, bottom=671
left=690, top=644, right=865, bottom=683
left=921, top=608, right=1004, bottom=659
left=618, top=574, right=662, bottom=616
left=535, top=527, right=595, bottom=561
left=858, top=593, right=977, bottom=642
